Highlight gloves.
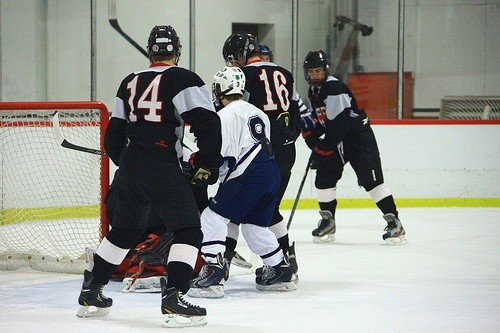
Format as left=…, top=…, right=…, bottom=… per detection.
left=309, top=146, right=333, bottom=169
left=303, top=127, right=319, bottom=152
left=191, top=154, right=224, bottom=187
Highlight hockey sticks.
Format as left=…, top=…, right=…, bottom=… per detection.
left=108, top=0, right=148, bottom=59
left=51, top=110, right=108, bottom=156
left=285, top=148, right=313, bottom=230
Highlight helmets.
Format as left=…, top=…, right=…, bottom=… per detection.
left=222, top=31, right=260, bottom=60
left=213, top=66, right=246, bottom=96
left=148, top=24, right=181, bottom=57
left=304, top=50, right=329, bottom=87
left=259, top=44, right=272, bottom=63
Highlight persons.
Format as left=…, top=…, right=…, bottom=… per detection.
left=257, top=44, right=274, bottom=63
left=208, top=31, right=300, bottom=282
left=91, top=150, right=207, bottom=289
left=79, top=24, right=210, bottom=317
left=301, top=50, right=410, bottom=240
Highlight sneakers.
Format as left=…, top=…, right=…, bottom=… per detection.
left=76, top=269, right=113, bottom=319
left=224, top=248, right=237, bottom=281
left=382, top=213, right=407, bottom=244
left=160, top=277, right=208, bottom=328
left=312, top=210, right=337, bottom=242
left=255, top=241, right=299, bottom=283
left=184, top=252, right=226, bottom=298
left=254, top=255, right=296, bottom=291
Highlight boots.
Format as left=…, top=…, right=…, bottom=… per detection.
left=121, top=234, right=168, bottom=292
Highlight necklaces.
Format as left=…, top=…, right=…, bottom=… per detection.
left=185, top=65, right=297, bottom=289
